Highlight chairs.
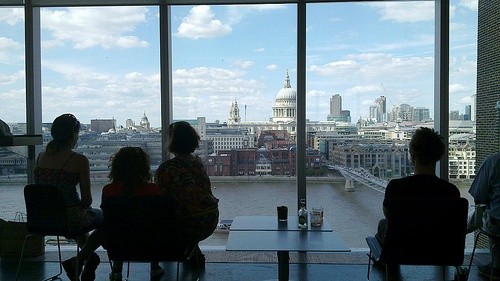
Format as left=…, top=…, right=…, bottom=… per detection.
left=14, top=183, right=95, bottom=281
left=365, top=197, right=468, bottom=281
left=100, top=195, right=198, bottom=281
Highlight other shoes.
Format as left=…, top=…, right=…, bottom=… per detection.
left=478, top=263, right=500, bottom=277
left=109, top=272, right=122, bottom=281
left=62, top=257, right=80, bottom=281
left=150, top=268, right=165, bottom=278
left=81, top=252, right=100, bottom=281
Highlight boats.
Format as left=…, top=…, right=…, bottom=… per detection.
left=213, top=222, right=232, bottom=234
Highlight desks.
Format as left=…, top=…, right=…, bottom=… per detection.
left=225, top=216, right=351, bottom=281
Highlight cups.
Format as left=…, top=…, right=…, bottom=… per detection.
left=309, top=207, right=323, bottom=227
left=277, top=206, right=288, bottom=222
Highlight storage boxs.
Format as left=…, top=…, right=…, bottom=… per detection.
left=0, top=222, right=45, bottom=256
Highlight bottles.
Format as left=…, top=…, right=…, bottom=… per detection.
left=298, top=199, right=308, bottom=229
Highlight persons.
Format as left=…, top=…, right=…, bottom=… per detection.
left=33, top=114, right=102, bottom=281
left=154, top=121, right=219, bottom=269
left=100, top=147, right=165, bottom=281
left=372, top=126, right=460, bottom=271
left=467, top=152, right=500, bottom=277
